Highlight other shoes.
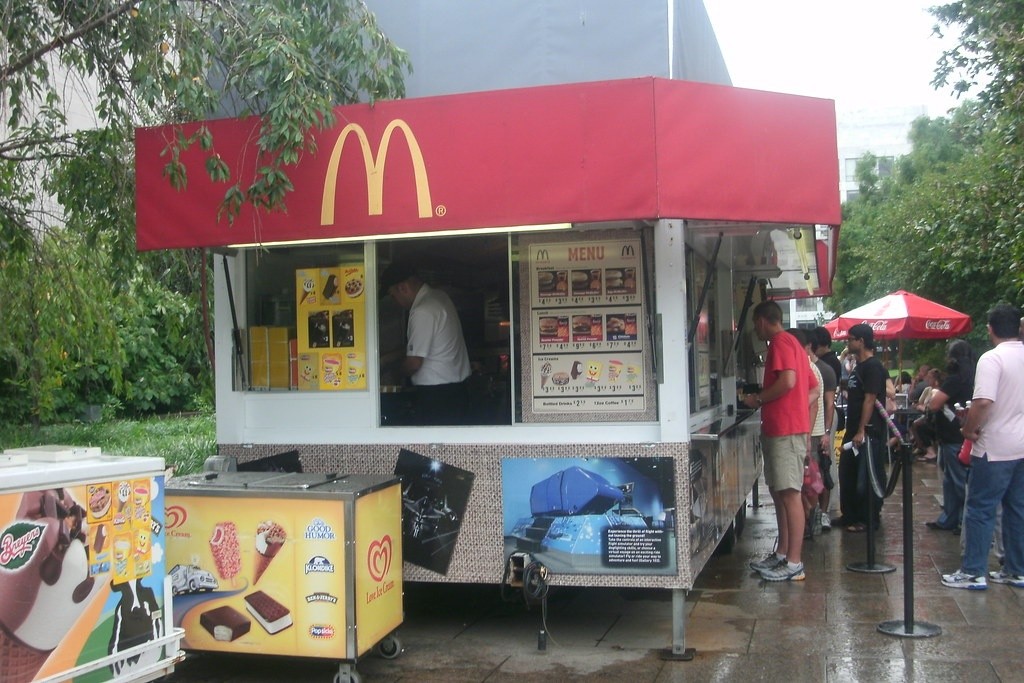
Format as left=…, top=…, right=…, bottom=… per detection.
left=830, top=516, right=855, bottom=527
left=847, top=521, right=876, bottom=533
left=912, top=448, right=925, bottom=455
left=820, top=511, right=831, bottom=532
left=925, top=520, right=952, bottom=531
left=809, top=507, right=823, bottom=541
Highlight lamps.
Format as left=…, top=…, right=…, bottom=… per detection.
left=792, top=234, right=813, bottom=296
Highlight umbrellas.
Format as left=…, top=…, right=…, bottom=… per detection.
left=824, top=289, right=972, bottom=394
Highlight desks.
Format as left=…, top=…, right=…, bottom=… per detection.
left=895, top=409, right=925, bottom=443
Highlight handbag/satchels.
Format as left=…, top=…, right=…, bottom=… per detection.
left=801, top=453, right=824, bottom=498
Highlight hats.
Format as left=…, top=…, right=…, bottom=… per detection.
left=380, top=259, right=414, bottom=296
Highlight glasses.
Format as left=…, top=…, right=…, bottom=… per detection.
left=847, top=336, right=865, bottom=342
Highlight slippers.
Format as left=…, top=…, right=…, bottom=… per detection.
left=918, top=452, right=939, bottom=462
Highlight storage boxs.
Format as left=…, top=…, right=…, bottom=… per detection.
left=249, top=326, right=298, bottom=390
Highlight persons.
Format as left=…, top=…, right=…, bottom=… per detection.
left=784, top=326, right=842, bottom=538
left=883, top=364, right=941, bottom=462
left=941, top=303, right=1024, bottom=589
left=929, top=340, right=981, bottom=535
left=839, top=346, right=852, bottom=391
left=743, top=300, right=819, bottom=581
left=384, top=259, right=473, bottom=425
left=831, top=324, right=887, bottom=532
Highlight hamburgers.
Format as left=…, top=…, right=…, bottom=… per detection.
left=540, top=315, right=593, bottom=335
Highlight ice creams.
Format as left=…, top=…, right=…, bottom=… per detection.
left=251, top=520, right=286, bottom=586
left=299, top=279, right=315, bottom=304
left=540, top=363, right=552, bottom=388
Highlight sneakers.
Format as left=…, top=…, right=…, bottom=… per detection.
left=750, top=551, right=786, bottom=572
left=941, top=568, right=986, bottom=590
left=760, top=558, right=806, bottom=581
left=988, top=564, right=1024, bottom=588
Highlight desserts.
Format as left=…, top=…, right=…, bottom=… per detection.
left=551, top=372, right=569, bottom=384
left=344, top=279, right=363, bottom=298
left=200, top=605, right=251, bottom=642
left=243, top=590, right=293, bottom=635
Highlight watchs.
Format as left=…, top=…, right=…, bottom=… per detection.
left=756, top=395, right=762, bottom=403
